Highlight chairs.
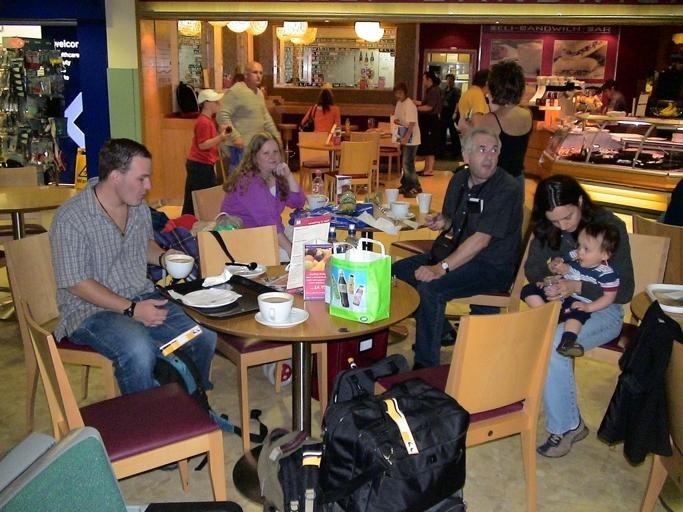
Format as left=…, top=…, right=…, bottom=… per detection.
left=192, top=184, right=227, bottom=222
left=0, top=167, right=37, bottom=191
left=299, top=122, right=401, bottom=193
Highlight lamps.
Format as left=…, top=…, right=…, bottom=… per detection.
left=227, top=21, right=247, bottom=34
left=248, top=21, right=268, bottom=35
left=284, top=21, right=307, bottom=38
left=178, top=20, right=201, bottom=36
left=355, top=21, right=380, bottom=40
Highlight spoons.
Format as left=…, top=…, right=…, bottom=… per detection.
left=225, top=262, right=258, bottom=270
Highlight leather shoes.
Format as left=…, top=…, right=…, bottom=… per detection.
left=412, top=329, right=457, bottom=350
left=413, top=361, right=425, bottom=369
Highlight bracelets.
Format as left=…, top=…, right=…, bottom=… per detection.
left=284, top=172, right=292, bottom=178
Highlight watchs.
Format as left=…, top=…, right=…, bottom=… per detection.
left=440, top=259, right=450, bottom=274
left=123, top=301, right=136, bottom=318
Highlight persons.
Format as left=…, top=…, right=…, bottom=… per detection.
left=301, top=87, right=341, bottom=138
left=180, top=62, right=282, bottom=216
left=413, top=62, right=531, bottom=181
left=391, top=128, right=525, bottom=371
left=520, top=222, right=621, bottom=358
left=392, top=83, right=422, bottom=193
left=217, top=131, right=306, bottom=262
left=601, top=79, right=627, bottom=112
left=524, top=174, right=634, bottom=459
left=48, top=137, right=235, bottom=471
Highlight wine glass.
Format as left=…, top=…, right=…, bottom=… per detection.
left=367, top=118, right=374, bottom=132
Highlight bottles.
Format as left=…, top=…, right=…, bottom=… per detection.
left=345, top=117, right=351, bottom=134
left=328, top=226, right=346, bottom=253
left=353, top=285, right=365, bottom=307
left=331, top=275, right=341, bottom=300
left=311, top=169, right=325, bottom=195
left=360, top=69, right=366, bottom=89
left=543, top=274, right=565, bottom=305
left=346, top=274, right=355, bottom=307
left=338, top=270, right=349, bottom=307
left=342, top=222, right=359, bottom=249
left=363, top=186, right=369, bottom=203
left=362, top=227, right=373, bottom=252
left=333, top=126, right=342, bottom=147
left=340, top=190, right=356, bottom=211
left=378, top=76, right=385, bottom=90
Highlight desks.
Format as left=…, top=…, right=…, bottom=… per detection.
left=279, top=101, right=396, bottom=121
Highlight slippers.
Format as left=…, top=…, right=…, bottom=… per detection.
left=416, top=171, right=433, bottom=176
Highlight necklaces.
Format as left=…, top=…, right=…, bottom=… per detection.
left=93, top=185, right=130, bottom=237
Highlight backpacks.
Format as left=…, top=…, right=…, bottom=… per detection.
left=278, top=378, right=469, bottom=512
left=152, top=349, right=268, bottom=471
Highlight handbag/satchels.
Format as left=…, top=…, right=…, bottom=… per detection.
left=330, top=354, right=410, bottom=407
left=329, top=238, right=391, bottom=324
left=258, top=428, right=311, bottom=512
left=197, top=225, right=281, bottom=279
left=432, top=236, right=457, bottom=260
left=303, top=119, right=314, bottom=132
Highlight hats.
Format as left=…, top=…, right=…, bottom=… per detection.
left=600, top=79, right=615, bottom=90
left=196, top=89, right=224, bottom=105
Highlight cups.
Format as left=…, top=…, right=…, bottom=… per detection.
left=416, top=192, right=432, bottom=215
left=550, top=98, right=554, bottom=105
left=546, top=99, right=550, bottom=106
left=554, top=99, right=558, bottom=106
left=257, top=290, right=294, bottom=322
left=384, top=189, right=400, bottom=209
left=165, top=254, right=195, bottom=280
left=540, top=99, right=545, bottom=105
left=306, top=194, right=329, bottom=212
left=389, top=202, right=410, bottom=217
left=536, top=99, right=540, bottom=105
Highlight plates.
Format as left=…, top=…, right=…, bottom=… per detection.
left=645, top=283, right=683, bottom=313
left=180, top=288, right=239, bottom=309
left=255, top=307, right=310, bottom=329
left=385, top=211, right=415, bottom=221
left=224, top=263, right=268, bottom=279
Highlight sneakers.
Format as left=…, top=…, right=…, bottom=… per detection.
left=404, top=188, right=422, bottom=198
left=397, top=185, right=407, bottom=194
left=556, top=344, right=584, bottom=356
left=537, top=415, right=589, bottom=457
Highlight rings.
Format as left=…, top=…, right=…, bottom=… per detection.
left=560, top=295, right=564, bottom=300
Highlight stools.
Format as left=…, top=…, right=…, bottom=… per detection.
left=276, top=124, right=298, bottom=164
